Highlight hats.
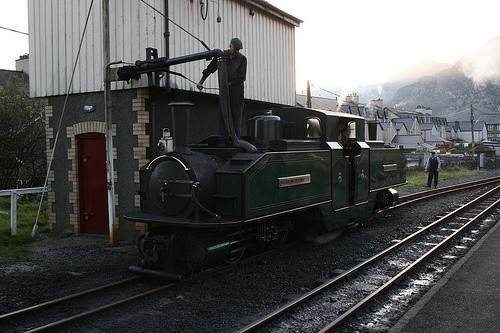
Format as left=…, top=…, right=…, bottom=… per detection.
left=230, top=37, right=243, bottom=49
left=430, top=152, right=435, bottom=154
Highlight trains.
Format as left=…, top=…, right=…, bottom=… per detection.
left=103, top=47, right=411, bottom=285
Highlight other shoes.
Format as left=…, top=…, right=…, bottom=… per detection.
left=425, top=185, right=431, bottom=188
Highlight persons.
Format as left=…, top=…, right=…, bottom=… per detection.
left=197, top=38, right=247, bottom=139
left=424, top=152, right=440, bottom=188
